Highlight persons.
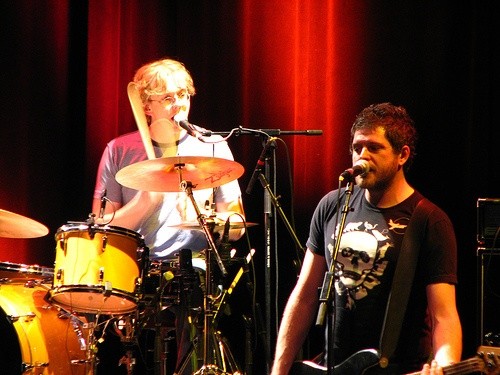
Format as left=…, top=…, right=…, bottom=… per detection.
left=271, top=103, right=464, bottom=375
left=93, top=59, right=247, bottom=275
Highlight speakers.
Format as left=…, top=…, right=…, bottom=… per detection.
left=477, top=253, right=500, bottom=356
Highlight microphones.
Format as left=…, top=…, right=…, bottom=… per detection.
left=338, top=159, right=370, bottom=182
left=99, top=199, right=107, bottom=219
left=245, top=138, right=271, bottom=195
left=221, top=221, right=232, bottom=269
left=174, top=114, right=202, bottom=141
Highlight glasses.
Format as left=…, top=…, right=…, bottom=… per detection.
left=150, top=93, right=186, bottom=106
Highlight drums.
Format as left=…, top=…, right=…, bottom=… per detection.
left=0, top=278, right=96, bottom=375
left=139, top=260, right=208, bottom=326
left=47, top=220, right=148, bottom=314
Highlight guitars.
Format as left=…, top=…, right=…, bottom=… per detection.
left=289, top=331, right=500, bottom=375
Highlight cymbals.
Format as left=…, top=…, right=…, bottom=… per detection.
left=115, top=156, right=244, bottom=192
left=167, top=217, right=259, bottom=233
left=0, top=208, right=49, bottom=239
